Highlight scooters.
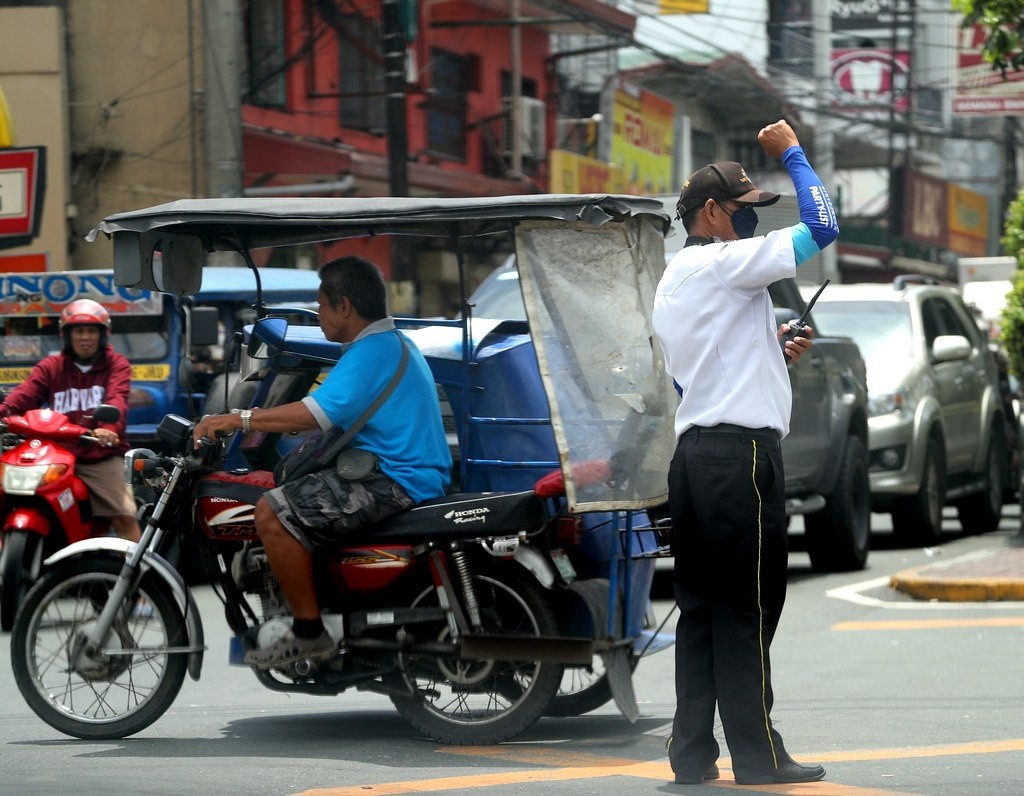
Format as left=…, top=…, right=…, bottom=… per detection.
left=0, top=387, right=159, bottom=629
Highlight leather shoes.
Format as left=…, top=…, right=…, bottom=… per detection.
left=673, top=761, right=721, bottom=785
left=735, top=757, right=827, bottom=785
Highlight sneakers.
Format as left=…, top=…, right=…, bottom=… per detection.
left=241, top=624, right=337, bottom=670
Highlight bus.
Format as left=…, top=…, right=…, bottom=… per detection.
left=2, top=245, right=364, bottom=471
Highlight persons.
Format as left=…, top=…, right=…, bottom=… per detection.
left=0, top=298, right=154, bottom=620
left=191, top=255, right=455, bottom=670
left=650, top=119, right=840, bottom=787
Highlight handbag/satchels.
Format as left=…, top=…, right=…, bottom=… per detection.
left=272, top=424, right=351, bottom=486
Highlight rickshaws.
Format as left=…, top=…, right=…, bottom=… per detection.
left=10, top=187, right=677, bottom=743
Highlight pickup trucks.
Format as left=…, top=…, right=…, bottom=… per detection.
left=462, top=236, right=881, bottom=593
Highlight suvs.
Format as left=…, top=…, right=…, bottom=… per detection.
left=790, top=277, right=1007, bottom=551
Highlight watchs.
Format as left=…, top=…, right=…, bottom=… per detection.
left=240, top=409, right=252, bottom=435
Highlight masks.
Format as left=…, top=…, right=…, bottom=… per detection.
left=717, top=201, right=759, bottom=239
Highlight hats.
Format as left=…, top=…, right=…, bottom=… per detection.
left=673, top=161, right=781, bottom=222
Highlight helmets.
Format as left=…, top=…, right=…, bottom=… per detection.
left=58, top=298, right=112, bottom=357
